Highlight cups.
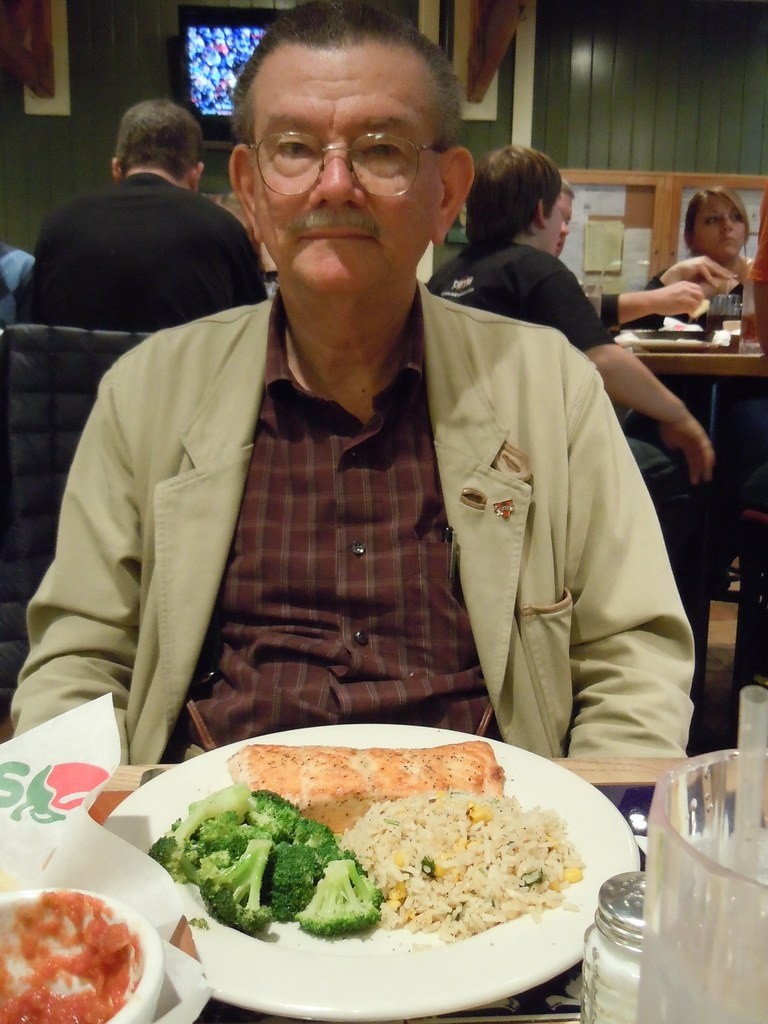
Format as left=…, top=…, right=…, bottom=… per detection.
left=634, top=745, right=768, bottom=1024
left=705, top=293, right=741, bottom=351
left=578, top=284, right=604, bottom=318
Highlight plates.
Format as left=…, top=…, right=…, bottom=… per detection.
left=613, top=333, right=719, bottom=351
left=100, top=724, right=642, bottom=1024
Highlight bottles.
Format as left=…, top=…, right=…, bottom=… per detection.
left=578, top=870, right=647, bottom=1024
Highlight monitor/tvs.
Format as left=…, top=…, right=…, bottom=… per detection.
left=177, top=4, right=279, bottom=149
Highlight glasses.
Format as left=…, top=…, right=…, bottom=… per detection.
left=249, top=132, right=447, bottom=198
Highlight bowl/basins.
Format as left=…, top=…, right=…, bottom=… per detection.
left=0, top=887, right=167, bottom=1024
left=632, top=330, right=712, bottom=341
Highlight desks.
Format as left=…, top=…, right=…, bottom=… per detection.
left=627, top=350, right=768, bottom=756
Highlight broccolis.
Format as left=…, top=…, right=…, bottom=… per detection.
left=148, top=784, right=386, bottom=937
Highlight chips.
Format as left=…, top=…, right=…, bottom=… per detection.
left=689, top=299, right=710, bottom=318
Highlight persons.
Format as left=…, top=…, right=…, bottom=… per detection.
left=739, top=187, right=768, bottom=513
left=624, top=186, right=768, bottom=589
left=554, top=181, right=704, bottom=325
left=10, top=2, right=697, bottom=758
left=427, top=145, right=715, bottom=589
left=18, top=98, right=267, bottom=334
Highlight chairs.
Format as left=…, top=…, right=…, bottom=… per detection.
left=0, top=325, right=153, bottom=739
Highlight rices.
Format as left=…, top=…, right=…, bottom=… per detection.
left=339, top=787, right=584, bottom=945
